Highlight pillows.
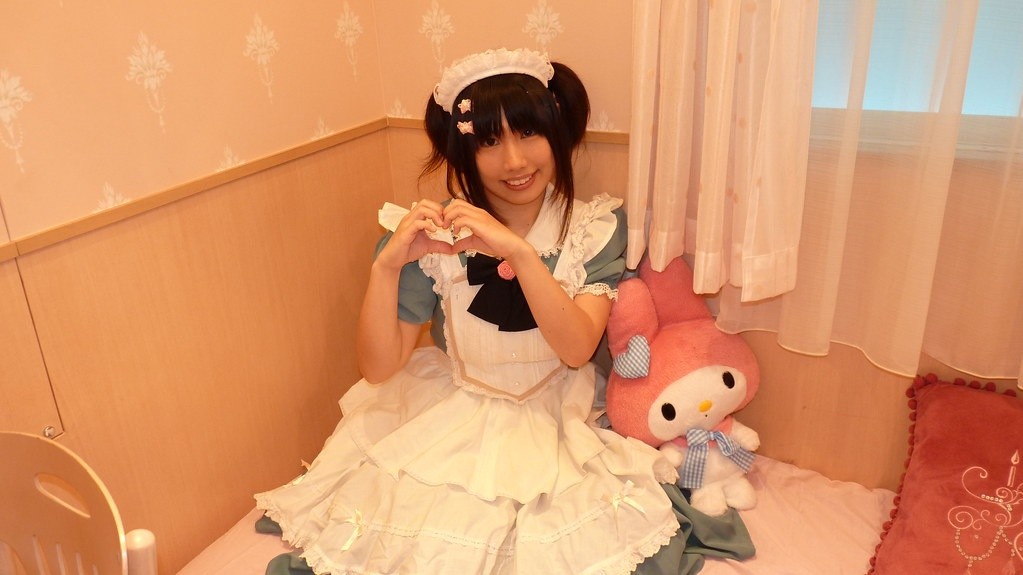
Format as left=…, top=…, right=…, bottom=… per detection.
left=866, top=372, right=1023, bottom=575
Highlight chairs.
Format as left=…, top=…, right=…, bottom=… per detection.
left=0, top=431, right=128, bottom=575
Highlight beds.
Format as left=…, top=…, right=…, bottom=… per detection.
left=167, top=428, right=898, bottom=575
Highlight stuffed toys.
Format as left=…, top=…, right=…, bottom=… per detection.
left=606, top=249, right=760, bottom=518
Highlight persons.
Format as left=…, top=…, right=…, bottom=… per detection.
left=255, top=50, right=757, bottom=575
